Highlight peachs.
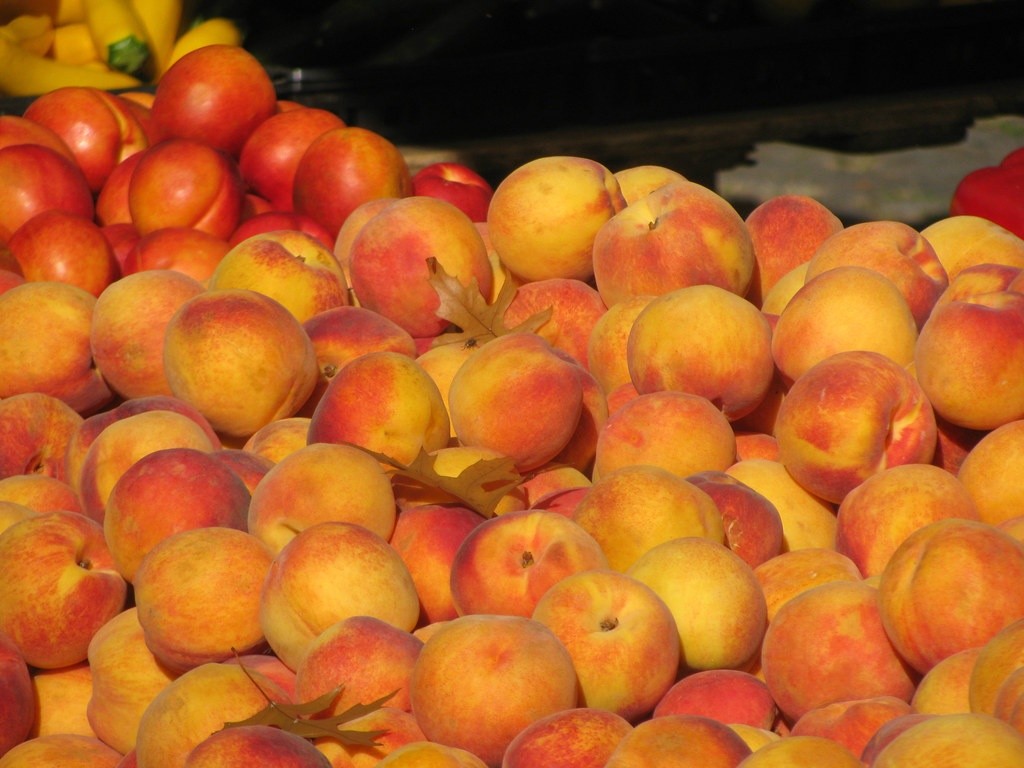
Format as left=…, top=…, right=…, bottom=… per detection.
left=0, top=44, right=490, bottom=299
left=0, top=157, right=1024, bottom=768
